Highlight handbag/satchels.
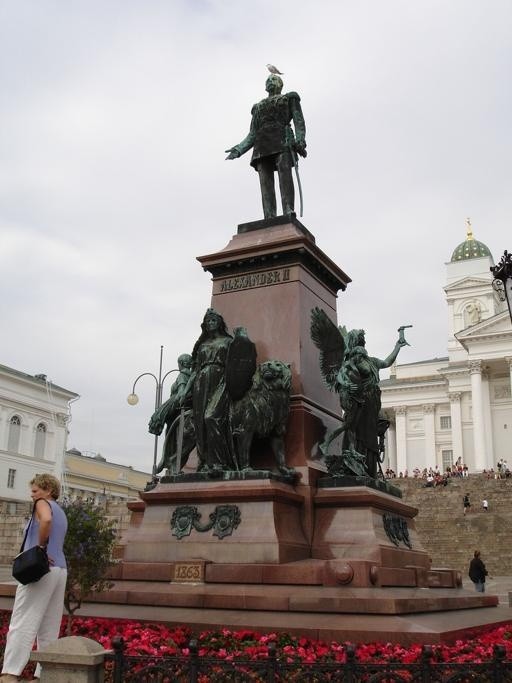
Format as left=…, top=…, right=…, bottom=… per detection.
left=12, top=546, right=51, bottom=586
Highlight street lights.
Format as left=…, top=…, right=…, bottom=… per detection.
left=127, top=344, right=180, bottom=482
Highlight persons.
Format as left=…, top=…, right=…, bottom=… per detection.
left=0, top=470, right=71, bottom=682
left=175, top=308, right=248, bottom=471
left=468, top=549, right=494, bottom=593
left=224, top=72, right=309, bottom=218
left=383, top=455, right=512, bottom=488
left=462, top=492, right=472, bottom=515
left=154, top=352, right=195, bottom=476
left=335, top=329, right=407, bottom=457
left=317, top=345, right=368, bottom=455
left=480, top=497, right=489, bottom=512
